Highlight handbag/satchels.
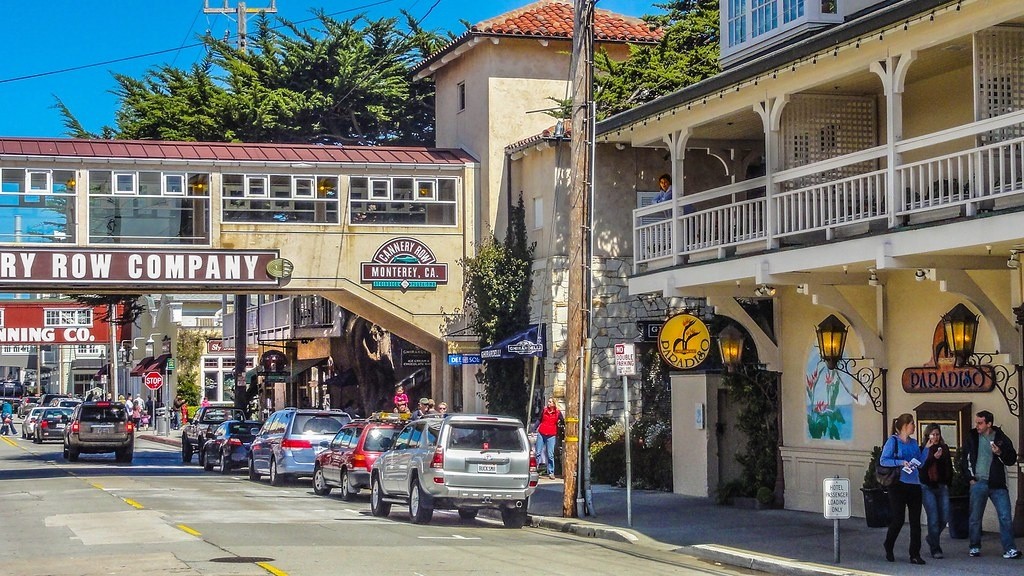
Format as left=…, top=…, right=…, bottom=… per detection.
left=875, top=436, right=900, bottom=488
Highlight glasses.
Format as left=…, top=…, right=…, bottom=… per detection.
left=440, top=408, right=445, bottom=410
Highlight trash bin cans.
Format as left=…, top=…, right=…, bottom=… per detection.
left=156, top=408, right=170, bottom=433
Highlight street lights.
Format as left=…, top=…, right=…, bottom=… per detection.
left=815, top=313, right=894, bottom=447
left=716, top=323, right=787, bottom=507
left=941, top=302, right=1023, bottom=537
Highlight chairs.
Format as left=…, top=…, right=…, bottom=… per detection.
left=101, top=411, right=116, bottom=418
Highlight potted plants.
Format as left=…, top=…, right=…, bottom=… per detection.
left=646, top=173, right=1024, bottom=273
left=861, top=445, right=891, bottom=529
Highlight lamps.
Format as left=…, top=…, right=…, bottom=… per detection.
left=813, top=312, right=884, bottom=414
left=754, top=284, right=776, bottom=297
left=941, top=300, right=1020, bottom=420
left=1006, top=249, right=1024, bottom=269
left=914, top=268, right=926, bottom=281
left=866, top=265, right=879, bottom=286
left=796, top=284, right=804, bottom=294
left=713, top=323, right=784, bottom=410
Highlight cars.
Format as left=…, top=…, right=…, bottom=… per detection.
left=200, top=419, right=265, bottom=475
left=48, top=397, right=83, bottom=412
left=31, top=409, right=70, bottom=443
left=34, top=394, right=68, bottom=410
left=16, top=396, right=40, bottom=419
left=20, top=406, right=51, bottom=440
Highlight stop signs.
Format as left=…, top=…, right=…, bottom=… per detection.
left=144, top=372, right=164, bottom=391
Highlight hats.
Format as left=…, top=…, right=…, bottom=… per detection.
left=419, top=398, right=429, bottom=404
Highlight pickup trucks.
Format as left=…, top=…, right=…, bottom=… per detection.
left=181, top=405, right=246, bottom=467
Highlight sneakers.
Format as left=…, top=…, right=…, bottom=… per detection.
left=968, top=547, right=981, bottom=557
left=1002, top=548, right=1022, bottom=559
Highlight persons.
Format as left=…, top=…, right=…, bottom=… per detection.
left=0, top=399, right=18, bottom=435
left=919, top=422, right=953, bottom=558
left=146, top=395, right=158, bottom=427
left=141, top=411, right=151, bottom=431
left=393, top=385, right=410, bottom=413
left=134, top=394, right=145, bottom=423
left=86, top=391, right=102, bottom=401
left=170, top=394, right=212, bottom=430
left=125, top=395, right=134, bottom=423
left=132, top=400, right=142, bottom=431
left=652, top=174, right=697, bottom=244
left=411, top=398, right=449, bottom=420
left=126, top=393, right=133, bottom=402
left=962, top=410, right=1023, bottom=559
left=119, top=395, right=127, bottom=405
left=53, top=398, right=60, bottom=407
left=531, top=397, right=566, bottom=480
left=879, top=414, right=937, bottom=565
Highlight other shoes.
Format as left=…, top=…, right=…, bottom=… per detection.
left=926, top=535, right=931, bottom=545
left=930, top=545, right=945, bottom=559
left=549, top=474, right=555, bottom=480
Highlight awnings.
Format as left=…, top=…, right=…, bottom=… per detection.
left=245, top=358, right=329, bottom=383
left=93, top=364, right=110, bottom=381
left=480, top=325, right=547, bottom=358
left=143, top=354, right=172, bottom=375
left=130, top=356, right=154, bottom=376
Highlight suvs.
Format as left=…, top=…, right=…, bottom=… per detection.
left=367, top=412, right=540, bottom=530
left=311, top=411, right=414, bottom=500
left=246, top=406, right=353, bottom=487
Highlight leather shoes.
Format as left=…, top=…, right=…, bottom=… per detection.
left=910, top=555, right=926, bottom=564
left=884, top=543, right=895, bottom=562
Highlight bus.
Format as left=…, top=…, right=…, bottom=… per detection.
left=64, top=401, right=135, bottom=464
